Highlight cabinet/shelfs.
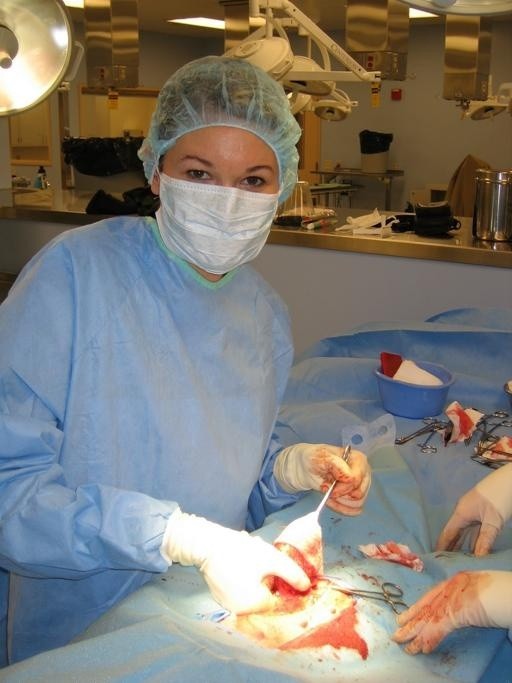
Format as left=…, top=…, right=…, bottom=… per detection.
left=8, top=86, right=70, bottom=165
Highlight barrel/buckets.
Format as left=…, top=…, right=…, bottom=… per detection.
left=472, top=169, right=512, bottom=241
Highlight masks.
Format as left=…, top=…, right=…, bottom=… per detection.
left=155, top=166, right=281, bottom=274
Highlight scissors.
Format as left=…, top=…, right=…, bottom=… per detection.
left=333, top=582, right=410, bottom=615
left=395, top=416, right=449, bottom=453
left=462, top=408, right=512, bottom=458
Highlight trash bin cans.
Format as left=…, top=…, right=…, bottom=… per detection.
left=359, top=129, right=393, bottom=174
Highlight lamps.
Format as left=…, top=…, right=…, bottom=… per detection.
left=466, top=73, right=507, bottom=120
left=1, top=0, right=84, bottom=117
left=216, top=0, right=383, bottom=124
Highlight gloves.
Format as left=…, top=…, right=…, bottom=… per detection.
left=273, top=443, right=371, bottom=517
left=434, top=461, right=512, bottom=557
left=159, top=507, right=311, bottom=615
left=390, top=570, right=512, bottom=655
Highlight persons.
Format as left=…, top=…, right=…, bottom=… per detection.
left=1, top=55, right=372, bottom=671
left=389, top=463, right=511, bottom=656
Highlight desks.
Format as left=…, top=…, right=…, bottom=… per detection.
left=309, top=165, right=405, bottom=211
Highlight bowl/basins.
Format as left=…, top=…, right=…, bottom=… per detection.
left=374, top=359, right=457, bottom=420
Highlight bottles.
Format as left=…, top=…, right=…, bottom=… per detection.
left=37, top=166, right=47, bottom=190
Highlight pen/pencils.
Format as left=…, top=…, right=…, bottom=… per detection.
left=307, top=219, right=328, bottom=230
